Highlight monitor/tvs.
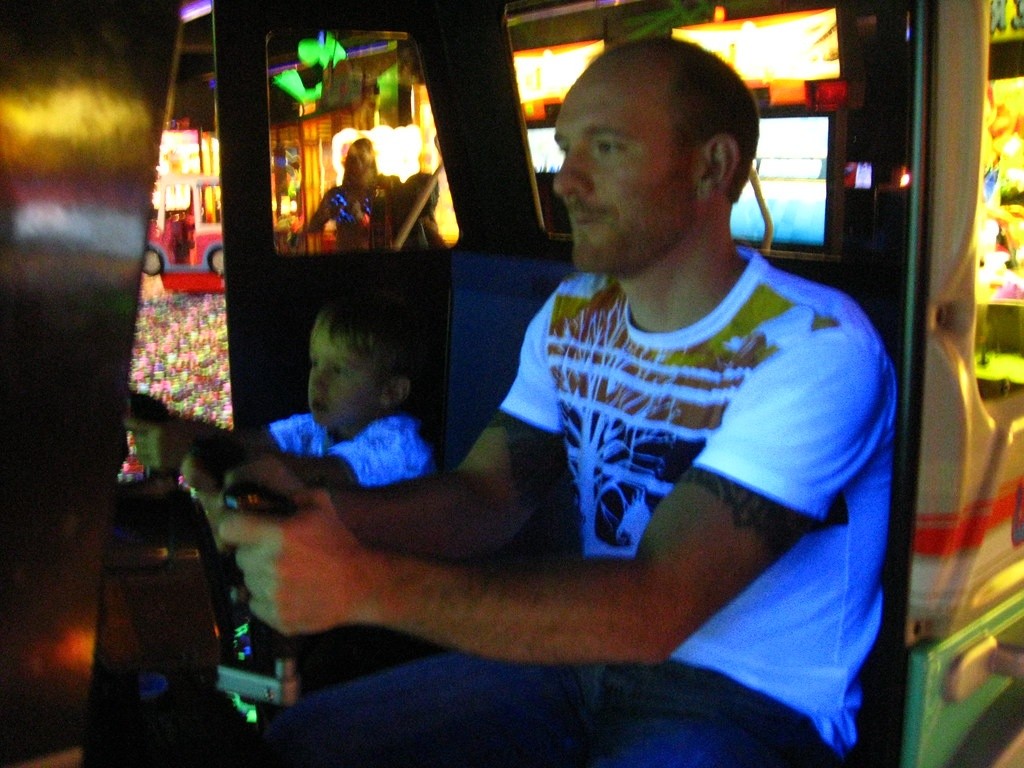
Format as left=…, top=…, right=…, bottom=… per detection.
left=729, top=108, right=834, bottom=259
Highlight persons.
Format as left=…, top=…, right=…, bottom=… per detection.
left=181, top=33, right=898, bottom=768
left=306, top=137, right=399, bottom=250
left=391, top=174, right=449, bottom=251
left=125, top=303, right=436, bottom=727
